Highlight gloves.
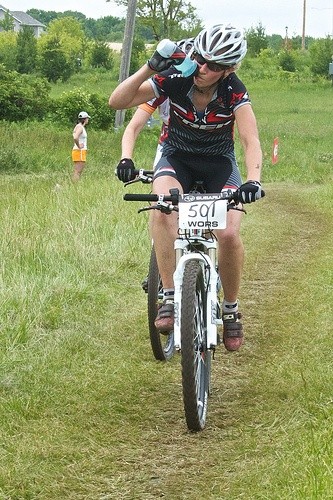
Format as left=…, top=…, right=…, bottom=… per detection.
left=234, top=180, right=262, bottom=205
left=116, top=158, right=136, bottom=183
left=149, top=43, right=183, bottom=72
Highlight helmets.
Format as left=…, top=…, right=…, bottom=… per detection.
left=194, top=23, right=248, bottom=65
left=177, top=37, right=197, bottom=55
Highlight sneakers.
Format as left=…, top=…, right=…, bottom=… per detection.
left=154, top=303, right=174, bottom=331
left=222, top=309, right=243, bottom=351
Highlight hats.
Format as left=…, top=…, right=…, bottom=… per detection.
left=78, top=112, right=91, bottom=118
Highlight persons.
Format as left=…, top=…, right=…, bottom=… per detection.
left=71, top=111, right=91, bottom=186
left=117, top=37, right=195, bottom=292
left=108, top=22, right=262, bottom=350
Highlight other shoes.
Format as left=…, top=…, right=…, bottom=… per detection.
left=142, top=274, right=162, bottom=290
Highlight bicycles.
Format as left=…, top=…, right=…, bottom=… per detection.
left=113, top=167, right=179, bottom=362
left=122, top=188, right=266, bottom=432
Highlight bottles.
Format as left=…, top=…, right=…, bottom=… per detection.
left=156, top=39, right=199, bottom=79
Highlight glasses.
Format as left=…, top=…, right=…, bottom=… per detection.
left=194, top=52, right=234, bottom=72
